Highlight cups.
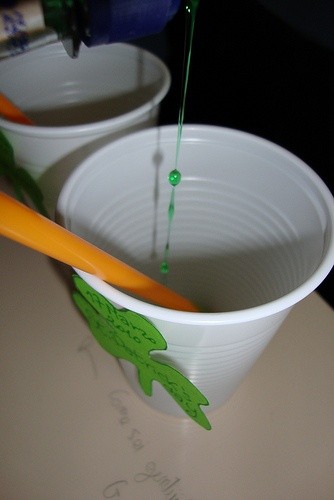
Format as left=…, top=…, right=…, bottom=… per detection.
left=51, top=121, right=334, bottom=419
left=0, top=41, right=173, bottom=223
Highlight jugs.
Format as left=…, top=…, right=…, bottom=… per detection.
left=53, top=0, right=181, bottom=57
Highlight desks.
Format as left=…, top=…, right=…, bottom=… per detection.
left=1, top=183, right=334, bottom=500
